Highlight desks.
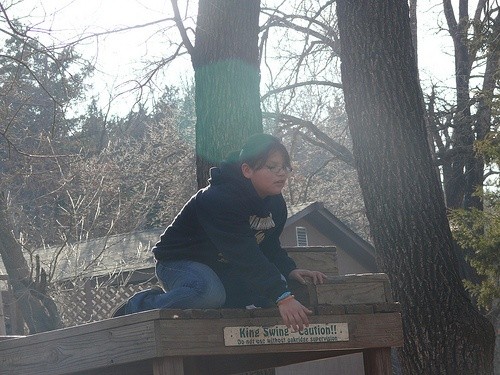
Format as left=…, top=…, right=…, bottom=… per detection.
left=0, top=308, right=406, bottom=375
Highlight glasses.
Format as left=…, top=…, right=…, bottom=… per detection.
left=261, top=163, right=293, bottom=176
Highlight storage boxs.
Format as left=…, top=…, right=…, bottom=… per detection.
left=279, top=246, right=394, bottom=315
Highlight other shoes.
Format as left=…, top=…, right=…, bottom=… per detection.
left=112, top=286, right=163, bottom=317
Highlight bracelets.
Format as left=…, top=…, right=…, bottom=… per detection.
left=277, top=295, right=295, bottom=305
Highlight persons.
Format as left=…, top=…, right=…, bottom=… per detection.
left=109, top=134, right=329, bottom=333
